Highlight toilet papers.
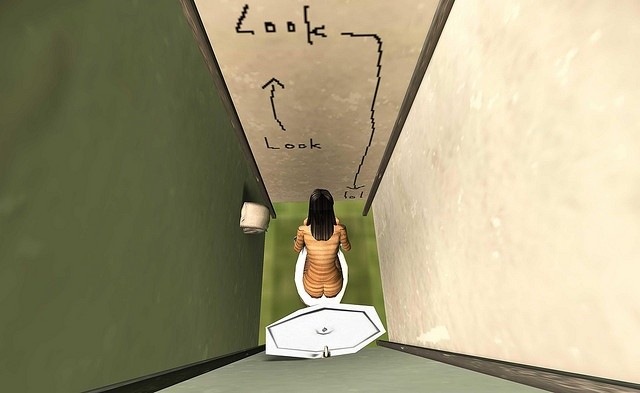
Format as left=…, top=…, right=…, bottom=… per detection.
left=243, top=228, right=265, bottom=235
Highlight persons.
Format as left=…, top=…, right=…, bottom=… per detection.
left=292, top=187, right=352, bottom=299
